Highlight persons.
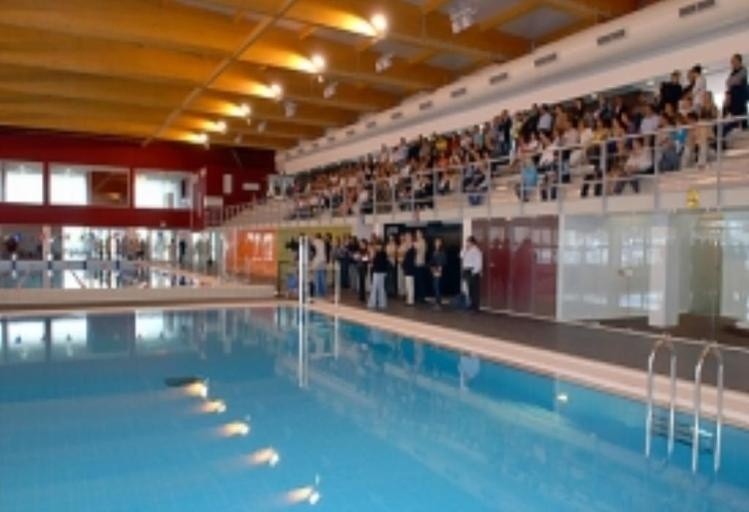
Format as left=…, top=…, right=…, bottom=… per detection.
left=69, top=234, right=187, bottom=268
left=285, top=229, right=485, bottom=316
left=263, top=54, right=749, bottom=222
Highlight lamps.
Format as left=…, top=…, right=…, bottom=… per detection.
left=316, top=72, right=339, bottom=100
left=438, top=0, right=475, bottom=36
left=367, top=34, right=394, bottom=74
